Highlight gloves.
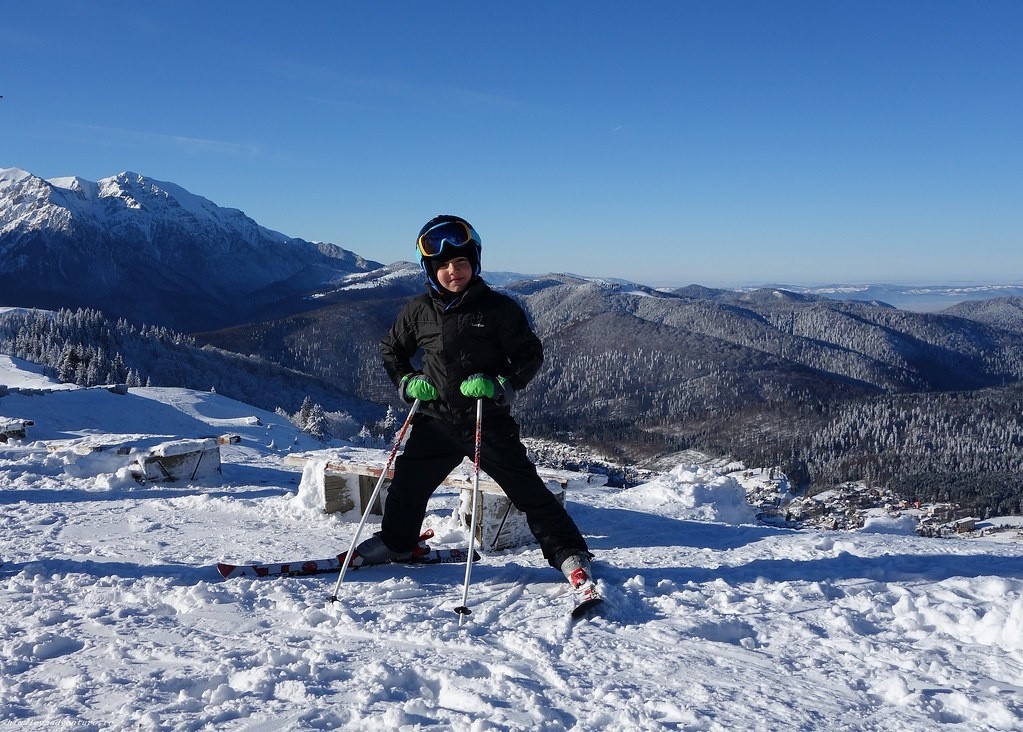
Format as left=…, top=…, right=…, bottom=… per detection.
left=461, top=373, right=516, bottom=406
left=398, top=372, right=440, bottom=406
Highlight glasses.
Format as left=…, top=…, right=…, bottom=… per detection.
left=415, top=221, right=481, bottom=269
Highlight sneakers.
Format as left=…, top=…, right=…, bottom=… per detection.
left=561, top=554, right=600, bottom=603
left=356, top=536, right=413, bottom=560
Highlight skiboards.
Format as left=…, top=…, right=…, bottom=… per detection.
left=216, top=541, right=605, bottom=621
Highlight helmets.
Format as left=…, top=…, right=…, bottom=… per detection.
left=415, top=214, right=482, bottom=291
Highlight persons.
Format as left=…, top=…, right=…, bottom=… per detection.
left=356, top=215, right=597, bottom=603
